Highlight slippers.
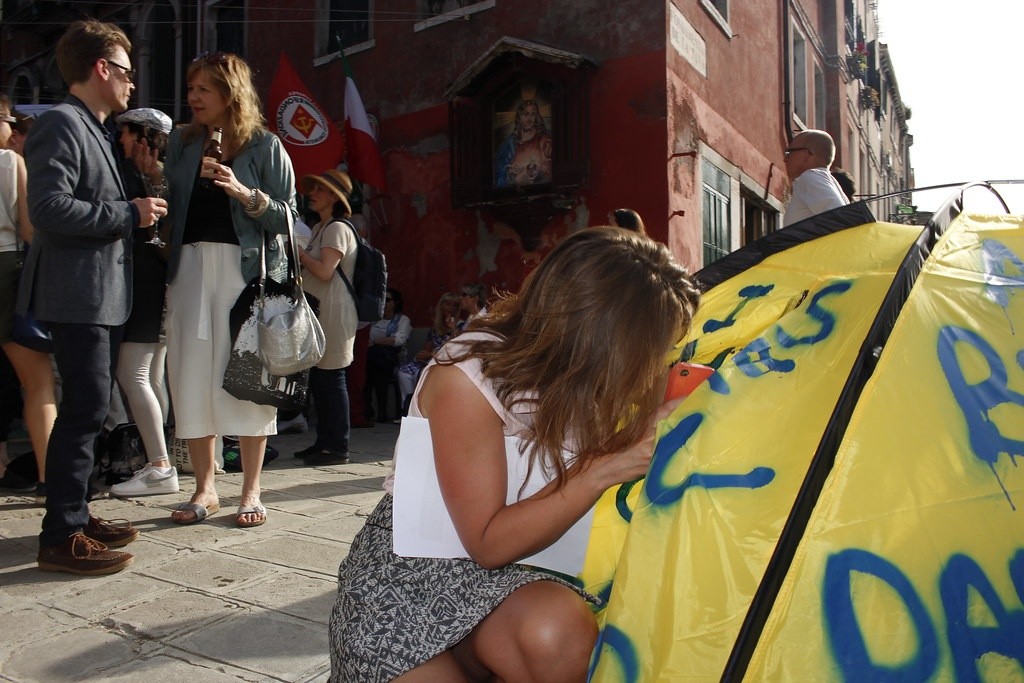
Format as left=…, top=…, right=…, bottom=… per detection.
left=172, top=503, right=220, bottom=524
left=236, top=506, right=267, bottom=527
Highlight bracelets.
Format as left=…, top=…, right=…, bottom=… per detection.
left=245, top=190, right=257, bottom=211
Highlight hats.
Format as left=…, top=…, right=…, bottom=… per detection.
left=302, top=168, right=353, bottom=218
left=115, top=107, right=173, bottom=134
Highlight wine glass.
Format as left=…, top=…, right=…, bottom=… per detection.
left=144, top=175, right=169, bottom=245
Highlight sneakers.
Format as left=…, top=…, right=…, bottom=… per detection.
left=109, top=463, right=179, bottom=496
left=37, top=532, right=134, bottom=575
left=85, top=516, right=139, bottom=547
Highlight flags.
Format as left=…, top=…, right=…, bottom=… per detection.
left=267, top=52, right=344, bottom=194
left=338, top=44, right=385, bottom=190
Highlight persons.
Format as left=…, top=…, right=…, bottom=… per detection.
left=130, top=50, right=296, bottom=524
left=608, top=208, right=646, bottom=233
left=348, top=289, right=411, bottom=428
left=295, top=169, right=356, bottom=463
left=783, top=129, right=856, bottom=227
left=393, top=284, right=485, bottom=427
left=329, top=226, right=697, bottom=682
left=0, top=96, right=312, bottom=505
left=21, top=20, right=168, bottom=576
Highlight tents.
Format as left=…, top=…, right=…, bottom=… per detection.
left=590, top=176, right=1024, bottom=683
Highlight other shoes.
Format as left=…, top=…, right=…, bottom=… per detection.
left=294, top=447, right=322, bottom=459
left=351, top=412, right=374, bottom=428
left=394, top=419, right=401, bottom=423
left=305, top=451, right=349, bottom=465
left=0, top=468, right=37, bottom=491
left=278, top=413, right=308, bottom=435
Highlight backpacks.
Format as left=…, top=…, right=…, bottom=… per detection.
left=320, top=217, right=388, bottom=321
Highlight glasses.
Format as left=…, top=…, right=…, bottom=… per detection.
left=784, top=147, right=813, bottom=159
left=90, top=59, right=136, bottom=79
left=1, top=115, right=17, bottom=126
left=20, top=112, right=39, bottom=121
left=387, top=296, right=394, bottom=302
left=462, top=292, right=472, bottom=297
left=192, top=50, right=229, bottom=73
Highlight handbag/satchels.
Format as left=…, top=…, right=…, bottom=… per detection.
left=222, top=275, right=320, bottom=408
left=257, top=289, right=325, bottom=375
left=368, top=344, right=396, bottom=369
left=12, top=267, right=55, bottom=353
left=106, top=424, right=278, bottom=485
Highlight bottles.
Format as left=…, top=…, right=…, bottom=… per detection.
left=196, top=127, right=223, bottom=189
